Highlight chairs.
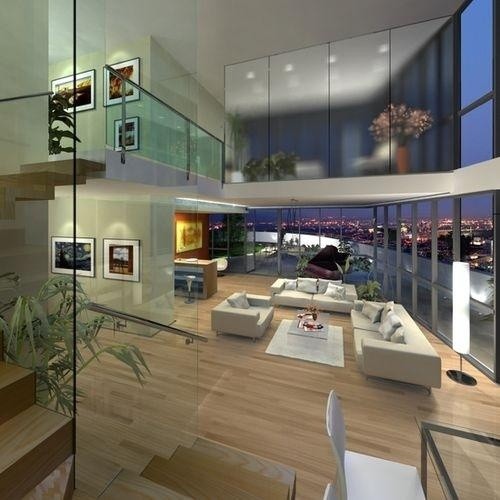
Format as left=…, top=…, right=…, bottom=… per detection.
left=322, top=388, right=426, bottom=500
left=211, top=258, right=228, bottom=276
left=323, top=483, right=338, bottom=500
left=211, top=292, right=274, bottom=343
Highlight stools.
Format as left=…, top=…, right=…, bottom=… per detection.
left=184, top=275, right=196, bottom=304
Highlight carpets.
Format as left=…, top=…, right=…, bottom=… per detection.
left=264, top=319, right=344, bottom=368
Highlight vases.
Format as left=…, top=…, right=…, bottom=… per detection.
left=396, top=147, right=410, bottom=173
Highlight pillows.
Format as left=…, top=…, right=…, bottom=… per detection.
left=284, top=280, right=297, bottom=289
left=297, top=277, right=318, bottom=294
left=380, top=301, right=394, bottom=323
left=378, top=311, right=404, bottom=340
left=362, top=298, right=384, bottom=323
left=390, top=326, right=407, bottom=344
left=323, top=282, right=345, bottom=300
left=226, top=291, right=250, bottom=309
left=316, top=277, right=343, bottom=294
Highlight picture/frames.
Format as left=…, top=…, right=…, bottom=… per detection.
left=114, top=116, right=140, bottom=152
left=103, top=57, right=140, bottom=107
left=51, top=236, right=96, bottom=279
left=51, top=69, right=96, bottom=114
left=103, top=238, right=140, bottom=282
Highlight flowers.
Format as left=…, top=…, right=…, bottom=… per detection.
left=368, top=104, right=434, bottom=147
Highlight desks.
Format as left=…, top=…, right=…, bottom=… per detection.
left=286, top=310, right=329, bottom=361
left=414, top=414, right=500, bottom=500
left=175, top=258, right=217, bottom=300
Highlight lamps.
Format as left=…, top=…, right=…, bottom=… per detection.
left=445, top=261, right=477, bottom=386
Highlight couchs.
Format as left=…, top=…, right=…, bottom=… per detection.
left=270, top=278, right=358, bottom=313
left=350, top=299, right=441, bottom=395
